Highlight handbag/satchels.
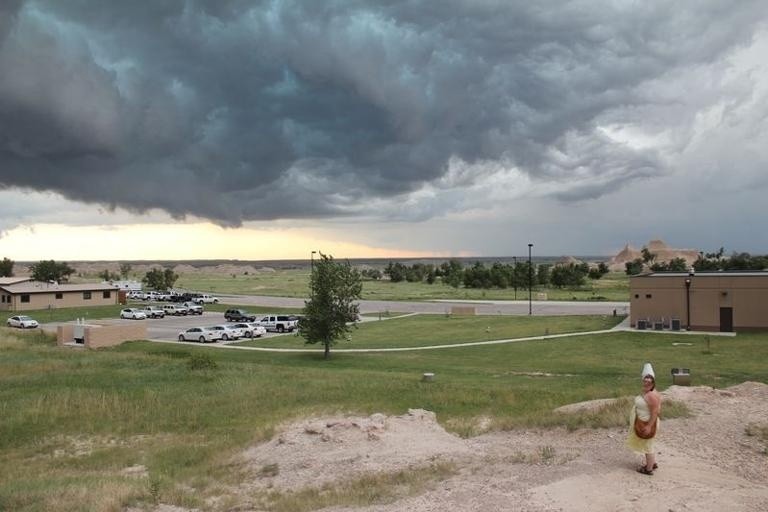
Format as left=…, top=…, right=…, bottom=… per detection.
left=635, top=415, right=656, bottom=438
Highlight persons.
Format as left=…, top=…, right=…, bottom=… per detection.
left=628, top=374, right=662, bottom=475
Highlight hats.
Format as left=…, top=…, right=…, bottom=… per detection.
left=641, top=363, right=655, bottom=381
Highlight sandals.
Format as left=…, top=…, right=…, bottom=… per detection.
left=637, top=463, right=658, bottom=475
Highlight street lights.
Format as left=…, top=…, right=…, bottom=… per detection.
left=527, top=243, right=534, bottom=314
left=311, top=251, right=317, bottom=300
left=512, top=257, right=517, bottom=300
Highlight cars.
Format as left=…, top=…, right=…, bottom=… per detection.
left=7, top=315, right=39, bottom=329
left=120, top=289, right=221, bottom=319
left=178, top=307, right=307, bottom=342
